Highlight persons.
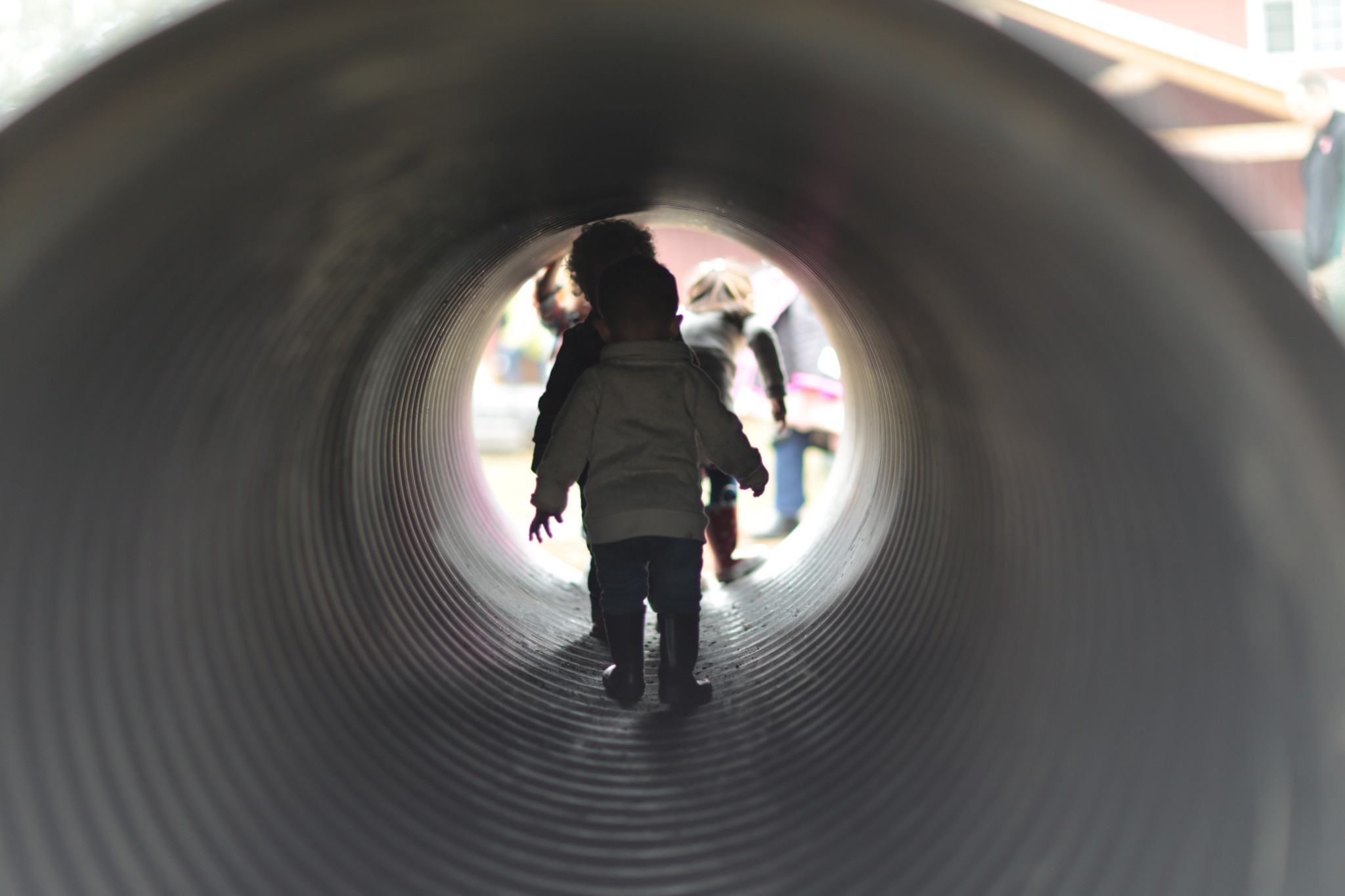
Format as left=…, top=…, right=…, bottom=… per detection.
left=536, top=253, right=590, bottom=361
left=675, top=253, right=788, bottom=585
left=532, top=216, right=732, bottom=644
left=524, top=255, right=769, bottom=712
left=746, top=260, right=843, bottom=541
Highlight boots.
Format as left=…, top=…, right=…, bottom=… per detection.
left=588, top=581, right=605, bottom=639
left=599, top=606, right=648, bottom=698
left=704, top=500, right=765, bottom=583
left=656, top=612, right=713, bottom=705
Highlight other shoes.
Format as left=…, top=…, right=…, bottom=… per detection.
left=753, top=518, right=797, bottom=544
